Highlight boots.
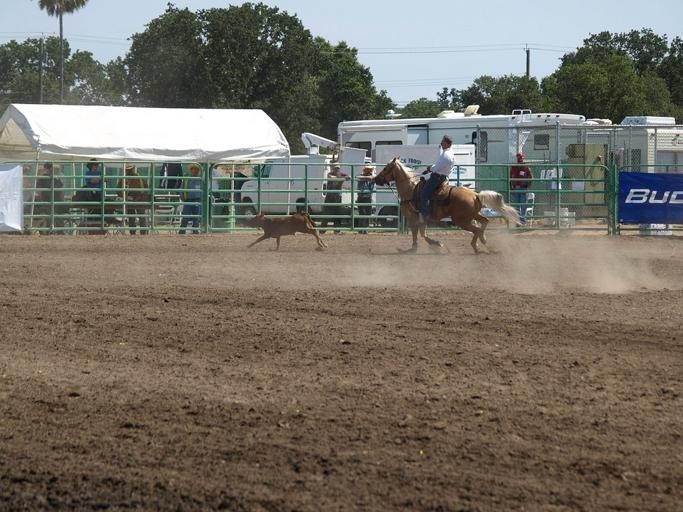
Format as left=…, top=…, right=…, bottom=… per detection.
left=421, top=200, right=430, bottom=216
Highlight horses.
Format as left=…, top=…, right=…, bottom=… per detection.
left=374, top=155, right=523, bottom=255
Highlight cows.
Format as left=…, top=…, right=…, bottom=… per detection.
left=246, top=212, right=327, bottom=252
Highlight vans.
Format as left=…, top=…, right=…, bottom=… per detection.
left=241, top=154, right=376, bottom=224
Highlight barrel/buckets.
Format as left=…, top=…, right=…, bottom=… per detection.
left=543, top=211, right=556, bottom=226
left=560, top=208, right=568, bottom=226
left=572, top=181, right=584, bottom=191
left=569, top=212, right=576, bottom=227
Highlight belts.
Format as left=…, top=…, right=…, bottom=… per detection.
left=511, top=185, right=527, bottom=189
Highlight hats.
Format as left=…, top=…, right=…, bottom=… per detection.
left=87, top=159, right=98, bottom=168
left=126, top=164, right=135, bottom=169
left=517, top=152, right=526, bottom=159
left=188, top=162, right=203, bottom=175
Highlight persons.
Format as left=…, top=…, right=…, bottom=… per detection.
left=24, top=162, right=65, bottom=235
left=179, top=164, right=201, bottom=234
left=418, top=134, right=455, bottom=216
left=319, top=165, right=352, bottom=234
left=355, top=166, right=376, bottom=234
left=84, top=156, right=101, bottom=188
left=510, top=154, right=532, bottom=224
left=115, top=163, right=148, bottom=235
